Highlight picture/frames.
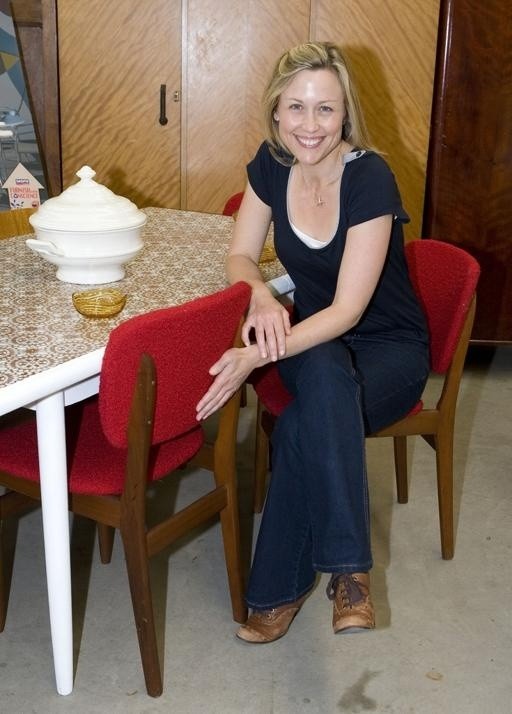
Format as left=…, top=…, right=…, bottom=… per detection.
left=0, top=201, right=295, bottom=696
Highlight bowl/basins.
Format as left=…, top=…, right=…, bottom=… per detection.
left=300, top=155, right=340, bottom=209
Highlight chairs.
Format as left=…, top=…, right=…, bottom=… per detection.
left=234, top=587, right=312, bottom=644
left=332, top=569, right=376, bottom=636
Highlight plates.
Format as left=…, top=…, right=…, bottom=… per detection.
left=40, top=0, right=441, bottom=239
left=420, top=0, right=512, bottom=373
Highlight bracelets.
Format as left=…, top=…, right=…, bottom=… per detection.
left=73, top=289, right=128, bottom=320
left=255, top=245, right=278, bottom=264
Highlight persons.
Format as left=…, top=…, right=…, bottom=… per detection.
left=192, top=38, right=436, bottom=646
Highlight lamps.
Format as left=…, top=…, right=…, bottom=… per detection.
left=248, top=240, right=480, bottom=560
left=0, top=280, right=251, bottom=699
left=220, top=192, right=257, bottom=408
